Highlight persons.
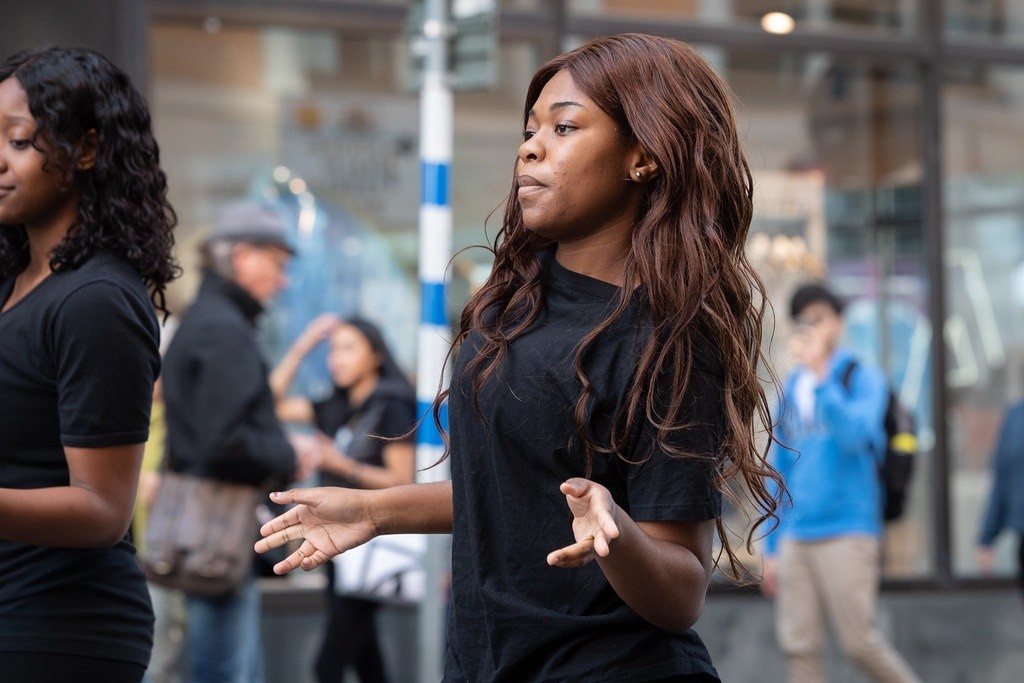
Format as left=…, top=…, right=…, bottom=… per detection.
left=0, top=42, right=182, bottom=683
left=975, top=397, right=1024, bottom=609
left=253, top=33, right=802, bottom=683
left=755, top=281, right=920, bottom=683
left=136, top=201, right=418, bottom=683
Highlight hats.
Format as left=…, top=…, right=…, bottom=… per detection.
left=197, top=201, right=291, bottom=250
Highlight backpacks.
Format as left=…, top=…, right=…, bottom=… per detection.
left=834, top=363, right=918, bottom=524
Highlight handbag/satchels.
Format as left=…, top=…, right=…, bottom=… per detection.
left=142, top=471, right=270, bottom=599
